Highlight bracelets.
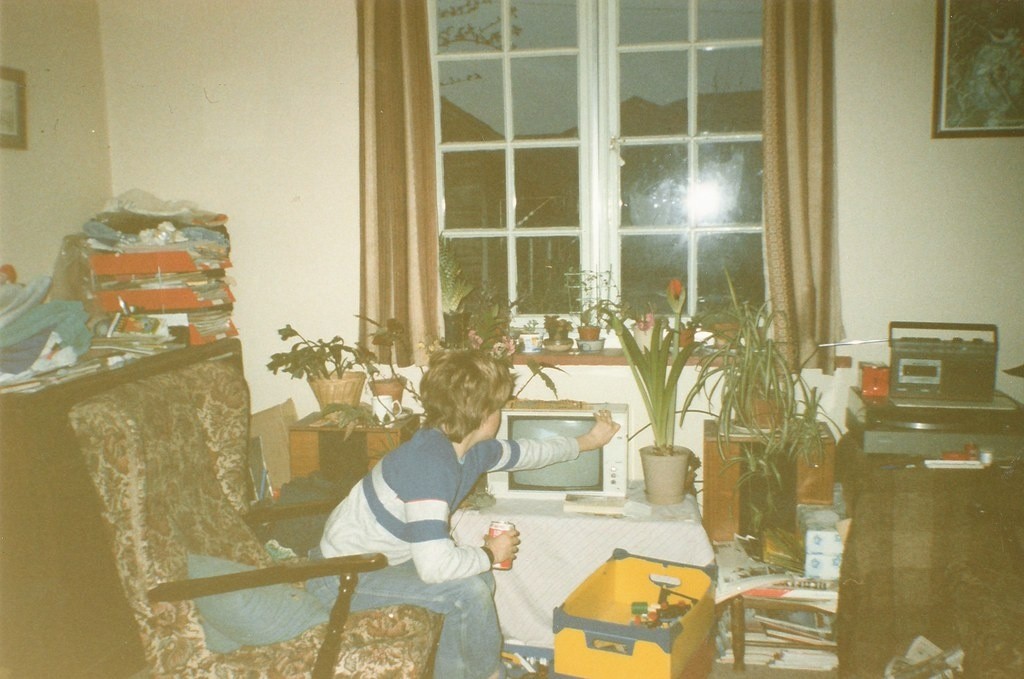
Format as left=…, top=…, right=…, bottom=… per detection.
left=480, top=546, right=494, bottom=564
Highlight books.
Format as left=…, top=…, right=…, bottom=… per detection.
left=711, top=528, right=838, bottom=649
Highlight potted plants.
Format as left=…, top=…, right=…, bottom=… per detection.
left=575, top=270, right=620, bottom=339
left=263, top=324, right=380, bottom=407
left=545, top=315, right=574, bottom=339
left=740, top=298, right=768, bottom=345
left=439, top=232, right=480, bottom=347
left=675, top=260, right=826, bottom=469
left=351, top=315, right=415, bottom=411
left=670, top=321, right=696, bottom=345
left=519, top=320, right=544, bottom=352
left=692, top=295, right=742, bottom=348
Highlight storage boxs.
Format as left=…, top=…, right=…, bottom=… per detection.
left=857, top=361, right=891, bottom=397
left=553, top=548, right=718, bottom=679
left=796, top=505, right=846, bottom=552
left=793, top=539, right=844, bottom=583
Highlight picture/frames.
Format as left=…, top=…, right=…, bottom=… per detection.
left=0, top=65, right=28, bottom=151
left=929, top=0, right=1024, bottom=139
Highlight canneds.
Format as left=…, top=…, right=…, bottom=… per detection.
left=488, top=521, right=514, bottom=570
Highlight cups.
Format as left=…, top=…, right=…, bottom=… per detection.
left=372, top=395, right=401, bottom=425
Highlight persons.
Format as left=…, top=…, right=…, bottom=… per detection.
left=305, top=346, right=621, bottom=679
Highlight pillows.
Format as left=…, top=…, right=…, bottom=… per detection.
left=183, top=553, right=332, bottom=656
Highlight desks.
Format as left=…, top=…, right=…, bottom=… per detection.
left=837, top=432, right=1024, bottom=679
left=700, top=418, right=836, bottom=543
left=458, top=482, right=712, bottom=658
left=0, top=337, right=245, bottom=679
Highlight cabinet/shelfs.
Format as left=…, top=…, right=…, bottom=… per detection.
left=287, top=411, right=420, bottom=479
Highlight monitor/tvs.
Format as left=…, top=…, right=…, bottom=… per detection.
left=488, top=404, right=630, bottom=500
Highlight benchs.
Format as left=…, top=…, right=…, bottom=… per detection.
left=69, top=360, right=446, bottom=679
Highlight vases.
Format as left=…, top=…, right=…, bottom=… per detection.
left=639, top=444, right=691, bottom=505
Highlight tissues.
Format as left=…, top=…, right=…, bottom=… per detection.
left=796, top=504, right=848, bottom=580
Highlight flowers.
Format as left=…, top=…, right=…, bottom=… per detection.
left=605, top=276, right=708, bottom=452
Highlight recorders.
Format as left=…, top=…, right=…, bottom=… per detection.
left=818, top=337, right=998, bottom=404
